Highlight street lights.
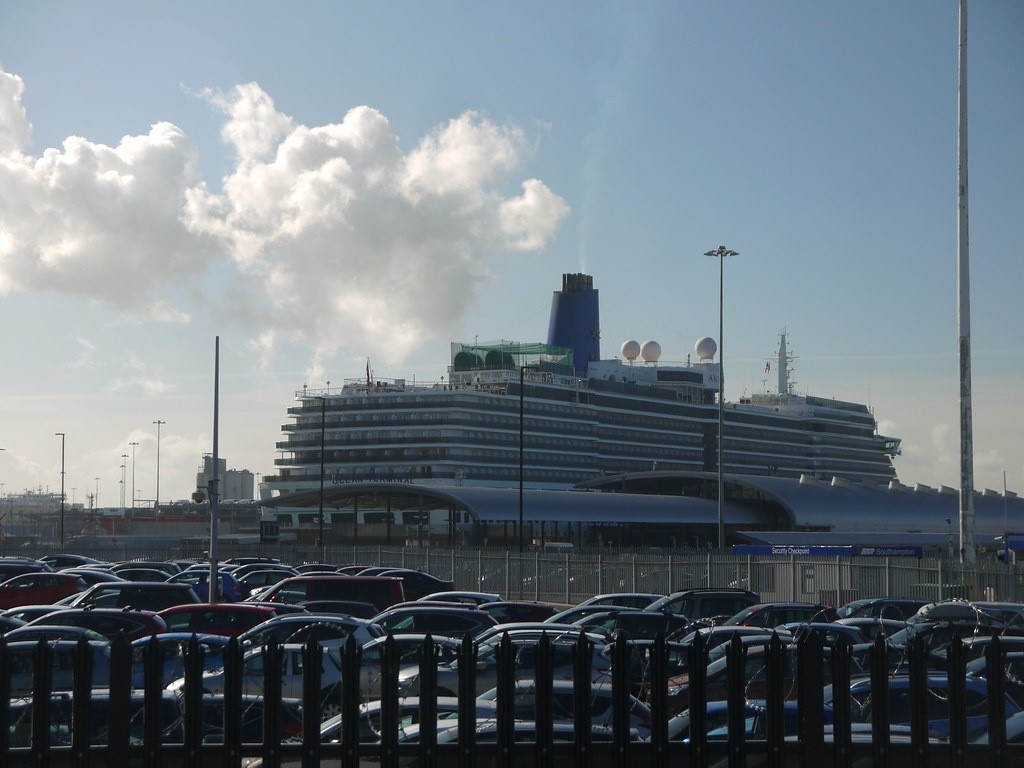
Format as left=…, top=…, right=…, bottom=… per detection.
left=54, top=433, right=66, bottom=548
left=516, top=365, right=542, bottom=596
left=701, top=245, right=742, bottom=554
left=118, top=454, right=131, bottom=511
left=94, top=478, right=101, bottom=508
left=317, top=395, right=326, bottom=548
left=152, top=419, right=166, bottom=509
left=129, top=442, right=139, bottom=511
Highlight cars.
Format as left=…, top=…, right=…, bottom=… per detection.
left=0, top=553, right=1021, bottom=768
